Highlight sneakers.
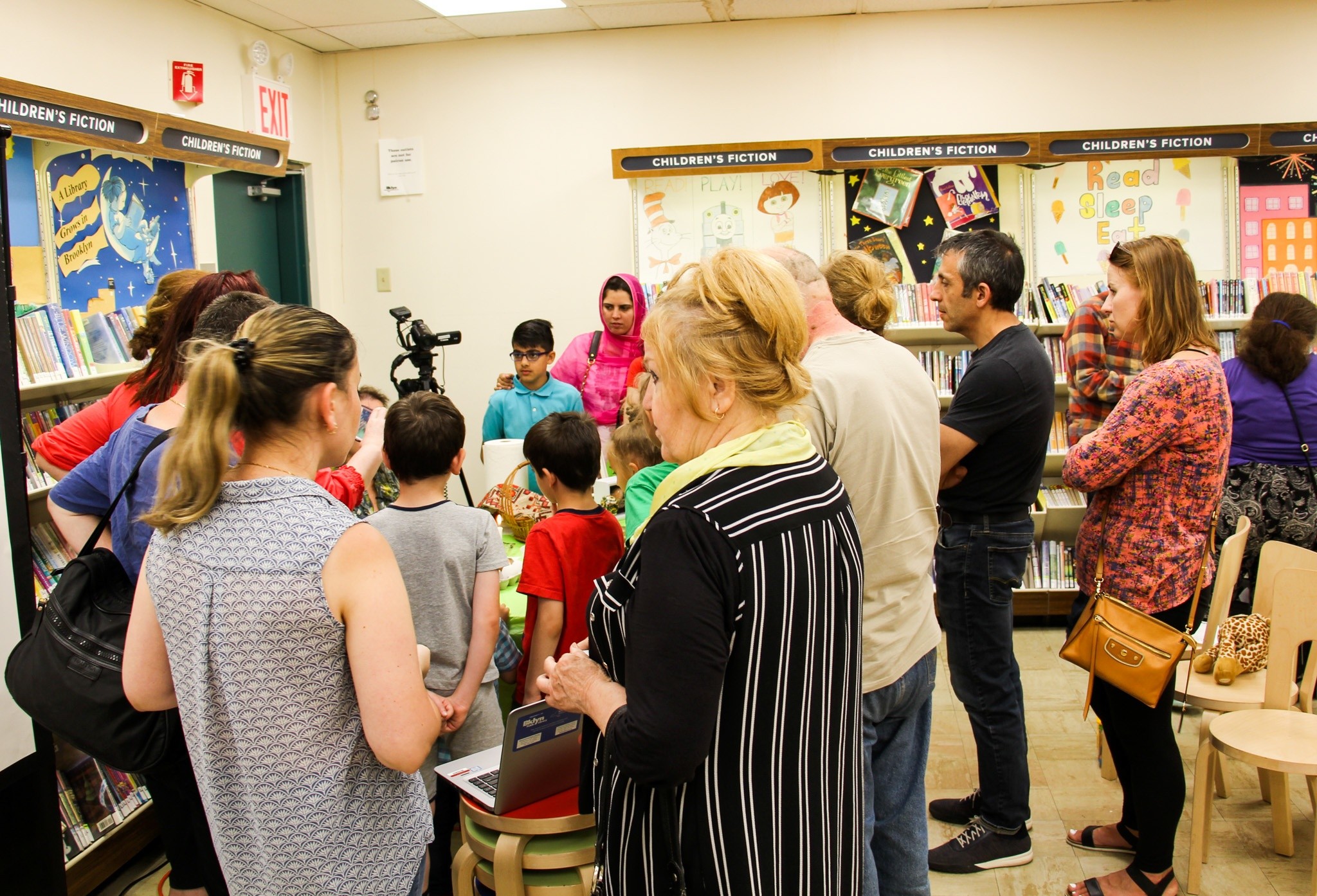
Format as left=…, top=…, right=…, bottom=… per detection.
left=928, top=787, right=1033, bottom=831
left=928, top=814, right=1033, bottom=874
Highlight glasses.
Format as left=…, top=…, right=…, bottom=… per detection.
left=1109, top=241, right=1132, bottom=260
left=510, top=351, right=548, bottom=361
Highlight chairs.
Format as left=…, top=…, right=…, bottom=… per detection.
left=1100, top=514, right=1317, bottom=896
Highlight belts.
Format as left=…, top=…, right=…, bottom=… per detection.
left=936, top=506, right=1029, bottom=528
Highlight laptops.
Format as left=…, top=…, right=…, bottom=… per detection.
left=435, top=699, right=583, bottom=815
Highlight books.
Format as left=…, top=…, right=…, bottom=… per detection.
left=891, top=272, right=1316, bottom=590
left=18, top=300, right=158, bottom=609
left=56, top=732, right=151, bottom=864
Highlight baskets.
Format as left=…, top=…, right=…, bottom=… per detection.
left=495, top=460, right=558, bottom=543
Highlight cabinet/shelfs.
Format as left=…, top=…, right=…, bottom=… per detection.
left=877, top=279, right=1317, bottom=615
left=11, top=284, right=161, bottom=896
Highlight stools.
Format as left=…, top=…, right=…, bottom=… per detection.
left=451, top=793, right=600, bottom=896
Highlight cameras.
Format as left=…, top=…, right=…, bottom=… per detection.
left=409, top=319, right=461, bottom=351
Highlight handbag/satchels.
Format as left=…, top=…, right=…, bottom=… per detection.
left=4, top=428, right=182, bottom=775
left=1058, top=591, right=1197, bottom=733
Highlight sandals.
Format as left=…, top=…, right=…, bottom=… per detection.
left=1064, top=862, right=1178, bottom=896
left=1066, top=821, right=1140, bottom=854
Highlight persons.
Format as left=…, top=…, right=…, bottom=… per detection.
left=31, top=269, right=680, bottom=895
left=929, top=229, right=1055, bottom=872
left=1058, top=236, right=1232, bottom=895
left=824, top=249, right=897, bottom=339
left=536, top=251, right=866, bottom=895
left=756, top=245, right=941, bottom=895
left=1197, top=292, right=1317, bottom=614
left=1061, top=291, right=1154, bottom=447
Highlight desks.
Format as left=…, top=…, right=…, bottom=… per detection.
left=487, top=510, right=626, bottom=721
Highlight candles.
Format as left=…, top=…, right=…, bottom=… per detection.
left=496, top=514, right=504, bottom=539
